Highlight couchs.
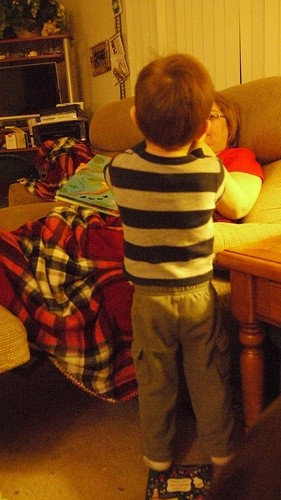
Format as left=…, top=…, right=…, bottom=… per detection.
left=0, top=76, right=281, bottom=401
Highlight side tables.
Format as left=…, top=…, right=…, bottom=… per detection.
left=222, top=232, right=281, bottom=433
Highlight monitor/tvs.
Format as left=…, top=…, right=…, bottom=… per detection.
left=0, top=62, right=62, bottom=117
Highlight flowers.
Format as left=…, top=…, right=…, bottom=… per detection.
left=0, top=0, right=61, bottom=32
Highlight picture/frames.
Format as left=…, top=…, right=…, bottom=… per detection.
left=89, top=40, right=112, bottom=77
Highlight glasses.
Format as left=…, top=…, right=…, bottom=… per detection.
left=208, top=112, right=226, bottom=121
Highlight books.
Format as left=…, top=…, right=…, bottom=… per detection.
left=54, top=154, right=119, bottom=218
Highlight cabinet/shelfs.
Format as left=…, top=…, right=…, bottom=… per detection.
left=0, top=34, right=86, bottom=157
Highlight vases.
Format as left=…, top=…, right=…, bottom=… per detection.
left=13, top=23, right=44, bottom=39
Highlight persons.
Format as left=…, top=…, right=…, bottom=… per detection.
left=103, top=54, right=237, bottom=471
left=76, top=91, right=264, bottom=224
